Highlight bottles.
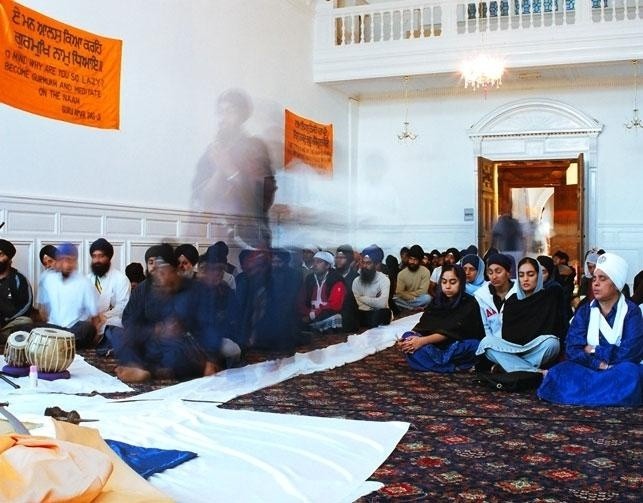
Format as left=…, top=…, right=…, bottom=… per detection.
left=30, top=366, right=37, bottom=388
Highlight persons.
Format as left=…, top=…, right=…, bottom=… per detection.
left=187, top=87, right=277, bottom=375
left=35, top=237, right=504, bottom=382
left=0, top=239, right=33, bottom=355
left=490, top=211, right=523, bottom=251
left=397, top=255, right=643, bottom=408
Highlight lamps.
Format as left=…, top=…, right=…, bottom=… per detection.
left=396, top=76, right=421, bottom=142
left=621, top=60, right=642, bottom=130
left=456, top=0, right=507, bottom=88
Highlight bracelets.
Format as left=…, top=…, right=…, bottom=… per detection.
left=226, top=170, right=240, bottom=180
